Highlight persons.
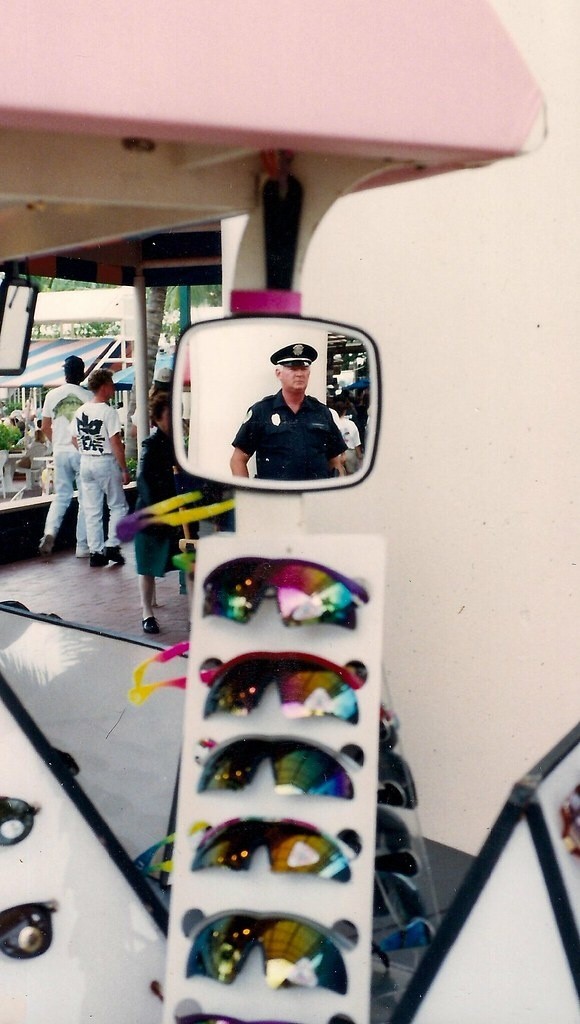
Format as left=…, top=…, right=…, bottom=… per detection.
left=40, top=353, right=97, bottom=557
left=0, top=402, right=50, bottom=469
left=230, top=344, right=347, bottom=481
left=130, top=392, right=206, bottom=633
left=327, top=387, right=369, bottom=476
left=72, top=370, right=133, bottom=567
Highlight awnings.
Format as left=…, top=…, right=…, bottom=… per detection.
left=2, top=336, right=126, bottom=390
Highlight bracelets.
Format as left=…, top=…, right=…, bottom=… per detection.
left=119, top=468, right=128, bottom=473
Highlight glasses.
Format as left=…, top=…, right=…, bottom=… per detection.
left=0, top=556, right=435, bottom=1024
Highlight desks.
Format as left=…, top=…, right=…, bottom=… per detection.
left=31, top=455, right=57, bottom=498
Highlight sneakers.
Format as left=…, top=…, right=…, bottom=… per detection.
left=76, top=547, right=90, bottom=557
left=89, top=552, right=109, bottom=566
left=39, top=534, right=55, bottom=561
left=104, top=546, right=124, bottom=564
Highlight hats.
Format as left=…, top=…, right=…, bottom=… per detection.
left=270, top=343, right=318, bottom=367
